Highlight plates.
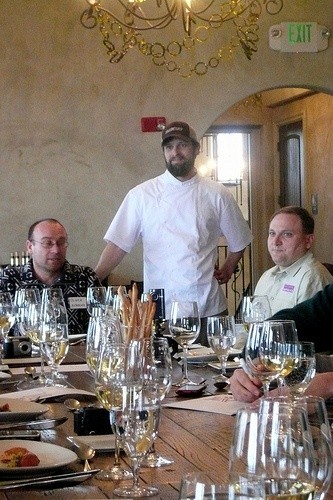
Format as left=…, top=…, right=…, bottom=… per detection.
left=207, top=361, right=242, bottom=371
left=65, top=434, right=124, bottom=452
left=173, top=348, right=242, bottom=363
left=0, top=398, right=50, bottom=421
left=68, top=334, right=86, bottom=342
left=0, top=440, right=78, bottom=475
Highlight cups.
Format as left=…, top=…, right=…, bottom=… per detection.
left=179, top=396, right=333, bottom=500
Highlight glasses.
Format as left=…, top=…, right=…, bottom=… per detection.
left=29, top=239, right=69, bottom=249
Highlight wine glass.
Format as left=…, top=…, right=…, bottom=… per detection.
left=0, top=286, right=316, bottom=500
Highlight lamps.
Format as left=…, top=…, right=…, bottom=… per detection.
left=269, top=22, right=330, bottom=53
left=81, top=0, right=283, bottom=78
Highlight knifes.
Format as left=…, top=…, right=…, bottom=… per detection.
left=0, top=416, right=69, bottom=431
left=0, top=468, right=103, bottom=491
left=0, top=473, right=93, bottom=492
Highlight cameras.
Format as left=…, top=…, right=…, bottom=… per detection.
left=3, top=335, right=33, bottom=359
left=72, top=405, right=127, bottom=436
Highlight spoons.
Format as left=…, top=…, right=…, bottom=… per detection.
left=64, top=398, right=81, bottom=412
left=24, top=367, right=39, bottom=380
left=214, top=379, right=229, bottom=389
left=76, top=444, right=96, bottom=472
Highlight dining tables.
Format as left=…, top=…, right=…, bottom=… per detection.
left=0, top=343, right=333, bottom=500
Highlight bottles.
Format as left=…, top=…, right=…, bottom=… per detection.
left=8, top=251, right=31, bottom=268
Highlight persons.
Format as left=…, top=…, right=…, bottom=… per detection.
left=229, top=283, right=333, bottom=400
left=254, top=206, right=333, bottom=316
left=0, top=218, right=106, bottom=334
left=93, top=122, right=254, bottom=347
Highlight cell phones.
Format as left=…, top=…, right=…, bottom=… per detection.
left=176, top=385, right=207, bottom=394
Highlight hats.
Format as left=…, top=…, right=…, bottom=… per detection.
left=161, top=121, right=200, bottom=148
